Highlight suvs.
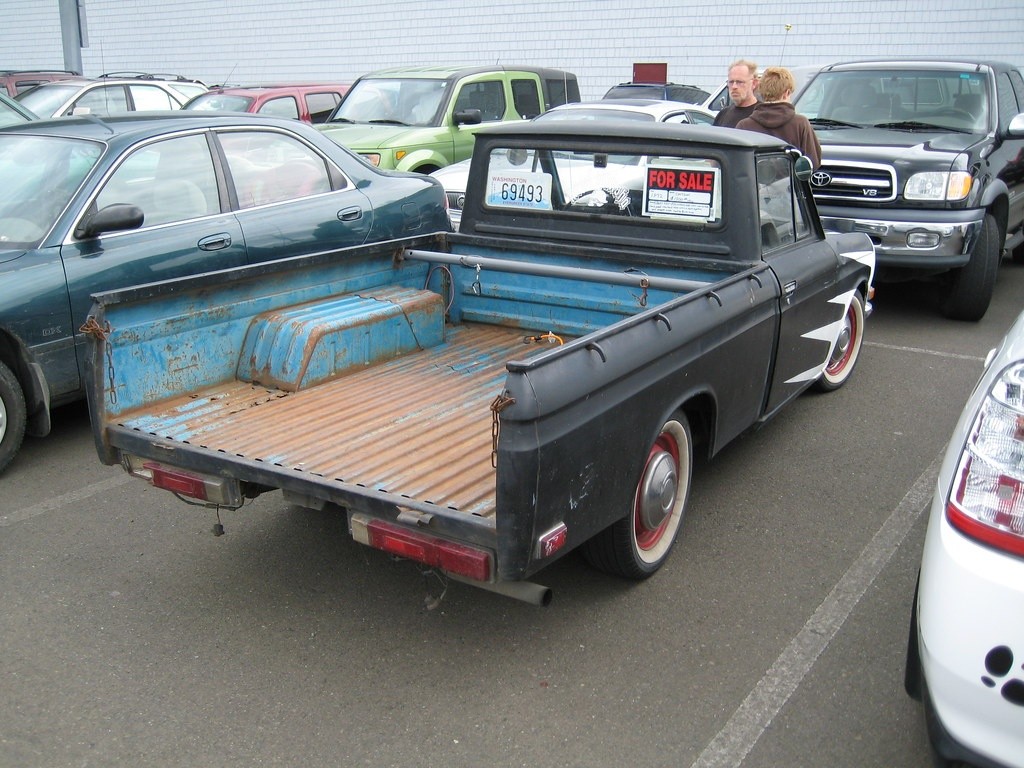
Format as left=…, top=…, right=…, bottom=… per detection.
left=789, top=56, right=1024, bottom=324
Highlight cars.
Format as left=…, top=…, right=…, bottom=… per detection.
left=0, top=61, right=823, bottom=235
left=0, top=108, right=456, bottom=480
left=905, top=315, right=1023, bottom=768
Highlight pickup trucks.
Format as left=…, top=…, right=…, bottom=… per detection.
left=80, top=120, right=876, bottom=607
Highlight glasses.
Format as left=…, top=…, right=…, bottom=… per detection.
left=725, top=80, right=747, bottom=86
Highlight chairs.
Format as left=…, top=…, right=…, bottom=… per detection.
left=469, top=90, right=501, bottom=121
left=142, top=177, right=207, bottom=225
left=830, top=81, right=880, bottom=122
left=952, top=92, right=983, bottom=120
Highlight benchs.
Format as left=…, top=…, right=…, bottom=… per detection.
left=867, top=92, right=953, bottom=119
left=228, top=158, right=323, bottom=207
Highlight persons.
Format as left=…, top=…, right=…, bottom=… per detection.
left=735, top=66, right=822, bottom=173
left=713, top=61, right=765, bottom=128
